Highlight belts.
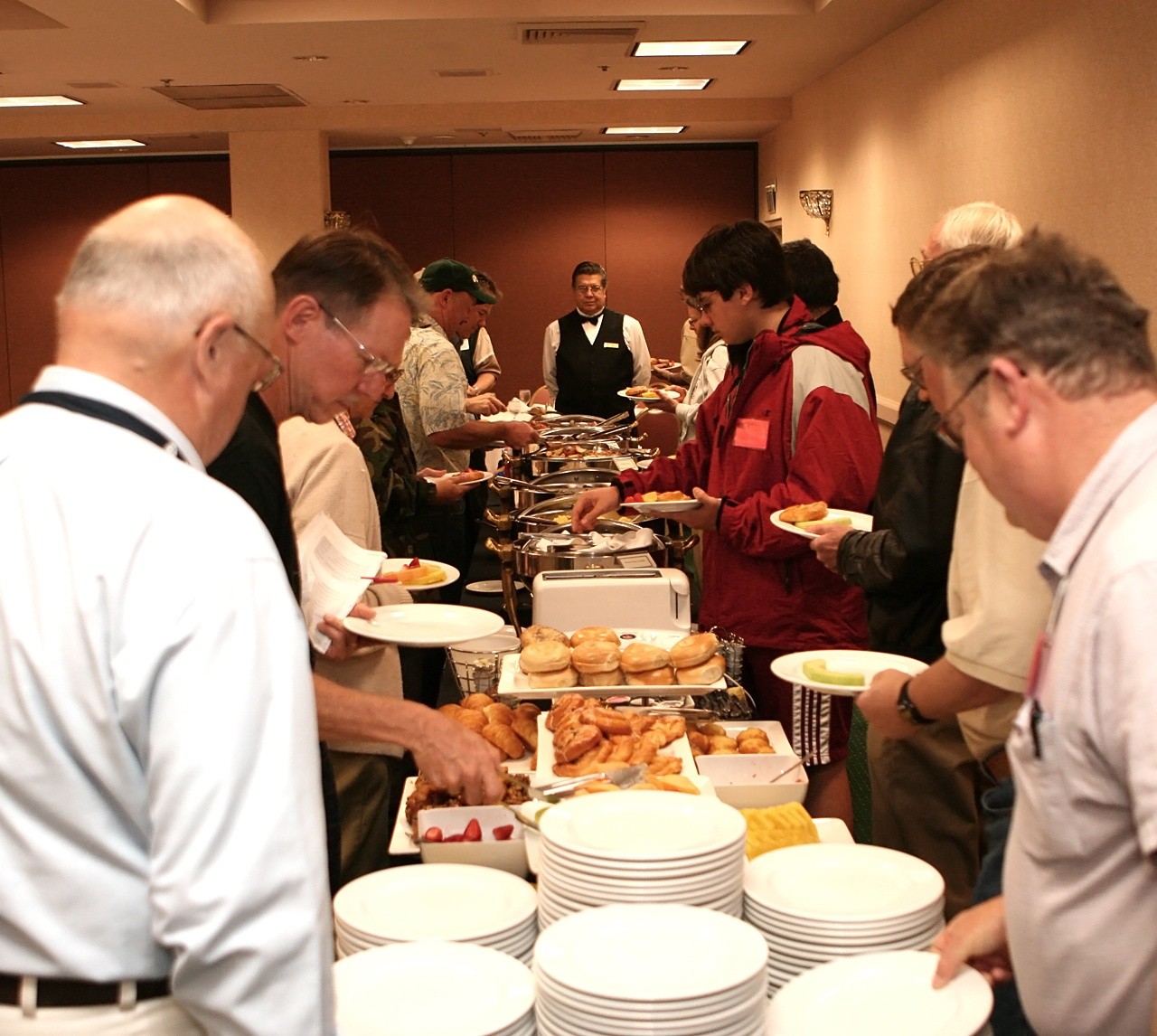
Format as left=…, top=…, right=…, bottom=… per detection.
left=0, top=973, right=171, bottom=1007
left=980, top=752, right=1012, bottom=784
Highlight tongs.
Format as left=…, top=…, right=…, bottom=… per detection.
left=595, top=411, right=629, bottom=426
left=530, top=762, right=648, bottom=796
left=496, top=770, right=560, bottom=832
left=571, top=424, right=631, bottom=441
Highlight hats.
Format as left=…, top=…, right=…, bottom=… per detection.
left=422, top=257, right=496, bottom=304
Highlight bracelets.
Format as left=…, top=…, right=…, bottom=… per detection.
left=668, top=372, right=673, bottom=384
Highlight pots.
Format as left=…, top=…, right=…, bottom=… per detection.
left=483, top=414, right=700, bottom=581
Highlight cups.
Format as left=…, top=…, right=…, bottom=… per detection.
left=448, top=625, right=527, bottom=697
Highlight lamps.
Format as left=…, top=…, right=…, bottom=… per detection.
left=324, top=209, right=351, bottom=232
left=797, top=189, right=833, bottom=238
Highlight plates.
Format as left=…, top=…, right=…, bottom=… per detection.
left=770, top=508, right=873, bottom=540
left=333, top=791, right=996, bottom=1036
left=371, top=558, right=460, bottom=590
left=342, top=603, right=505, bottom=647
left=423, top=471, right=493, bottom=485
left=465, top=581, right=524, bottom=592
left=497, top=654, right=727, bottom=699
left=770, top=650, right=929, bottom=696
left=651, top=363, right=681, bottom=371
left=388, top=710, right=808, bottom=855
left=617, top=388, right=681, bottom=402
left=619, top=498, right=722, bottom=513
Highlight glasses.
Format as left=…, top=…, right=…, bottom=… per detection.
left=195, top=322, right=285, bottom=392
left=574, top=285, right=603, bottom=296
left=318, top=302, right=395, bottom=375
left=931, top=364, right=1027, bottom=450
left=685, top=289, right=719, bottom=315
left=900, top=347, right=933, bottom=390
left=386, top=369, right=402, bottom=387
left=440, top=273, right=478, bottom=293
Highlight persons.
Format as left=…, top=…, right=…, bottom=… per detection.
left=0, top=192, right=542, bottom=1036
left=207, top=225, right=509, bottom=903
left=542, top=262, right=652, bottom=438
left=571, top=220, right=885, bottom=843
left=810, top=201, right=1062, bottom=924
left=925, top=227, right=1157, bottom=1036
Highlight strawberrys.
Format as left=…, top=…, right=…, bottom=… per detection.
left=426, top=818, right=514, bottom=843
left=373, top=577, right=397, bottom=583
left=452, top=467, right=484, bottom=480
left=408, top=557, right=421, bottom=569
left=623, top=493, right=644, bottom=502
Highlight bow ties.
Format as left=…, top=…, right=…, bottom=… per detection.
left=577, top=307, right=606, bottom=326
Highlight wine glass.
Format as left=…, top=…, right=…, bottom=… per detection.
left=519, top=389, right=530, bottom=406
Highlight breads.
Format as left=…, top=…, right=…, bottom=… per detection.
left=517, top=625, right=726, bottom=690
left=779, top=501, right=829, bottom=523
left=650, top=358, right=670, bottom=369
left=794, top=517, right=852, bottom=529
left=545, top=692, right=700, bottom=798
left=625, top=385, right=658, bottom=398
left=394, top=563, right=446, bottom=585
left=684, top=721, right=776, bottom=770
left=438, top=690, right=539, bottom=770
left=640, top=490, right=691, bottom=501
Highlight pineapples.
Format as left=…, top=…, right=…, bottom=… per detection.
left=734, top=802, right=821, bottom=861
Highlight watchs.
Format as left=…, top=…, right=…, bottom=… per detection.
left=897, top=677, right=941, bottom=726
left=472, top=385, right=481, bottom=395
